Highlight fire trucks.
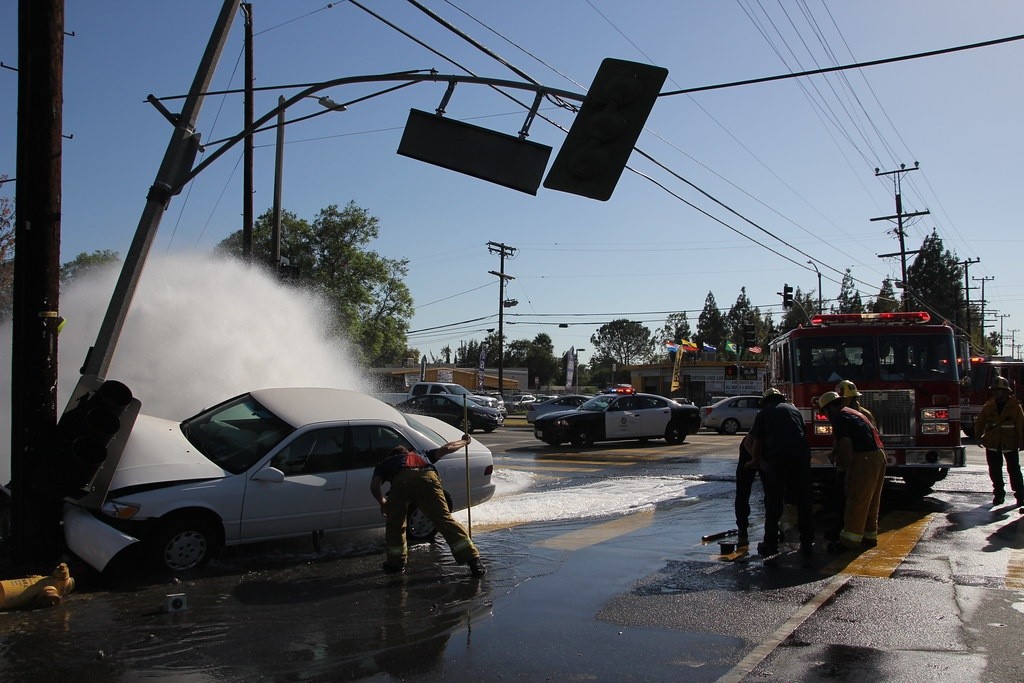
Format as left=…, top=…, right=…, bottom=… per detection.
left=762, top=310, right=967, bottom=487
left=938, top=356, right=1024, bottom=435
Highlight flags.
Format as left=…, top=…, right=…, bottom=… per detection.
left=665, top=339, right=761, bottom=356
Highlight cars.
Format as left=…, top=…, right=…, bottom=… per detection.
left=395, top=394, right=504, bottom=433
left=471, top=391, right=608, bottom=423
left=534, top=384, right=702, bottom=448
left=672, top=396, right=763, bottom=435
left=65, top=388, right=494, bottom=579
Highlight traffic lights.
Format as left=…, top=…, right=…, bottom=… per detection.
left=281, top=257, right=303, bottom=288
left=37, top=376, right=141, bottom=509
left=742, top=323, right=756, bottom=348
left=784, top=286, right=793, bottom=309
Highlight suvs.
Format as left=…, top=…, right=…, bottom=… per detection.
left=406, top=381, right=498, bottom=409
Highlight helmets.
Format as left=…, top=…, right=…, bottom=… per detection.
left=835, top=380, right=862, bottom=397
left=758, top=388, right=785, bottom=403
left=818, top=392, right=847, bottom=411
left=989, top=376, right=1011, bottom=391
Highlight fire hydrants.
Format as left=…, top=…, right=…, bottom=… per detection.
left=0, top=563, right=76, bottom=610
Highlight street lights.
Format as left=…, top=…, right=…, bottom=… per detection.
left=576, top=349, right=585, bottom=395
left=807, top=259, right=821, bottom=315
left=271, top=94, right=346, bottom=281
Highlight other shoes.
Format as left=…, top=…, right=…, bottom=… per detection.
left=1017, top=499, right=1024, bottom=505
left=737, top=539, right=749, bottom=546
left=470, top=559, right=487, bottom=573
left=840, top=539, right=861, bottom=547
left=384, top=561, right=405, bottom=573
left=797, top=547, right=812, bottom=554
left=758, top=542, right=779, bottom=551
left=993, top=497, right=1004, bottom=503
left=862, top=539, right=878, bottom=545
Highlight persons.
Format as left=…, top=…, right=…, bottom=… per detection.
left=825, top=380, right=877, bottom=539
left=975, top=377, right=1024, bottom=506
left=371, top=434, right=486, bottom=576
left=818, top=391, right=887, bottom=552
left=734, top=388, right=815, bottom=554
